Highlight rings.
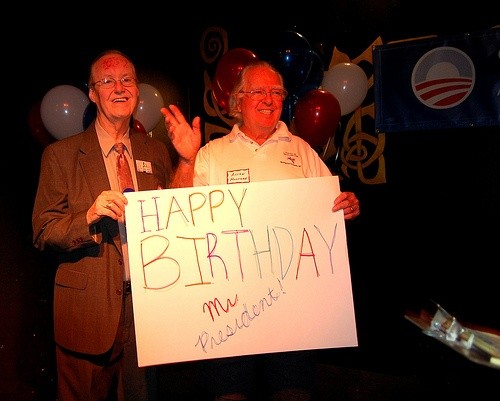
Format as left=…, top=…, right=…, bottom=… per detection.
left=106, top=201, right=111, bottom=206
left=351, top=208, right=354, bottom=211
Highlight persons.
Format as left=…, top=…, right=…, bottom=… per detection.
left=30, top=48, right=178, bottom=401
left=161, top=60, right=360, bottom=401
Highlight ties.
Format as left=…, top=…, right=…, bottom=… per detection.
left=113, top=143, right=135, bottom=193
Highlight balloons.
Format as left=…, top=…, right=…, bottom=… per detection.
left=132, top=119, right=146, bottom=135
left=211, top=29, right=369, bottom=154
left=132, top=83, right=164, bottom=132
left=39, top=83, right=90, bottom=141
left=83, top=103, right=134, bottom=130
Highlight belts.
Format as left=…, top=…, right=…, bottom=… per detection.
left=123, top=281, right=132, bottom=294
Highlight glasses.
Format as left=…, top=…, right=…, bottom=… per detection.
left=240, top=87, right=283, bottom=101
left=92, top=76, right=136, bottom=89
